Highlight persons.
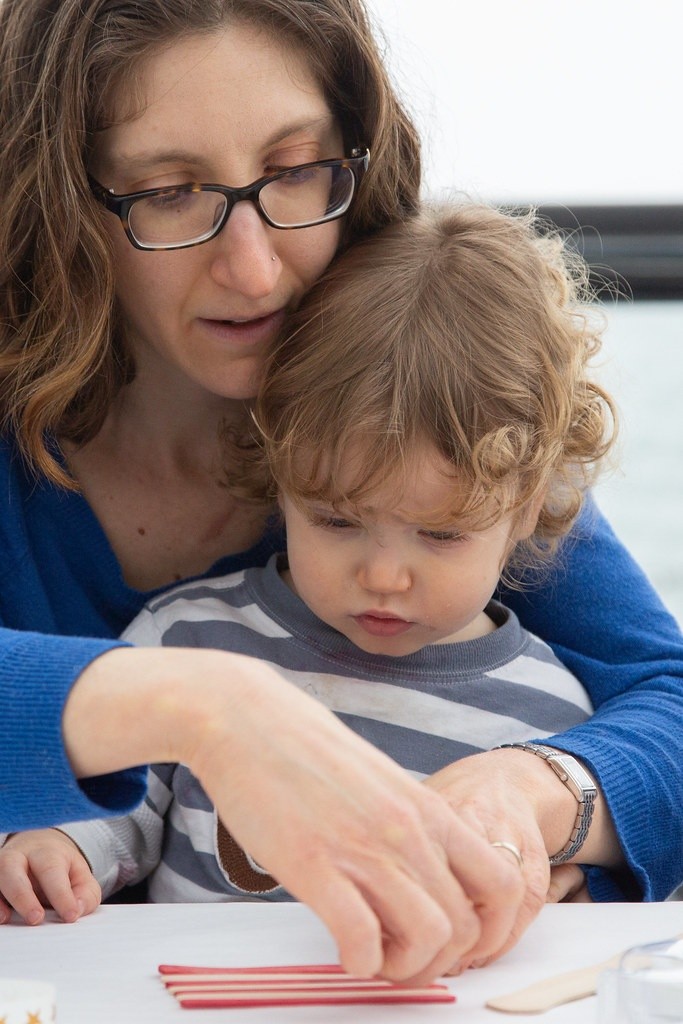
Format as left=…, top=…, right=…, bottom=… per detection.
left=1, top=1, right=682, bottom=983
left=0, top=202, right=626, bottom=926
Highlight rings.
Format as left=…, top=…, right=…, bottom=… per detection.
left=492, top=841, right=524, bottom=866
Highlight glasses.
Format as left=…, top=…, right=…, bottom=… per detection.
left=87, top=145, right=372, bottom=252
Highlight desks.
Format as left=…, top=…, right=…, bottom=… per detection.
left=1, top=902, right=683, bottom=1024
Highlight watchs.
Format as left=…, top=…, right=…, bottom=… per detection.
left=490, top=741, right=597, bottom=867
